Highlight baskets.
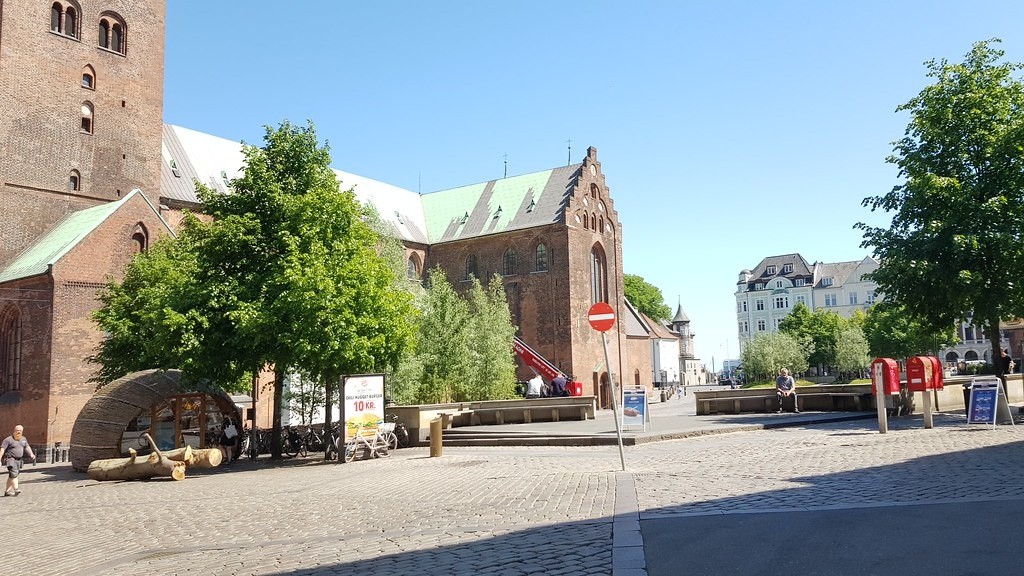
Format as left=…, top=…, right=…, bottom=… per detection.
left=382, top=423, right=395, bottom=432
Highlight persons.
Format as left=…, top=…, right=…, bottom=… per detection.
left=776, top=368, right=800, bottom=414
left=525, top=374, right=547, bottom=398
left=550, top=371, right=566, bottom=397
left=1000, top=347, right=1011, bottom=375
left=219, top=411, right=238, bottom=465
left=0, top=424, right=35, bottom=497
left=676, top=386, right=682, bottom=400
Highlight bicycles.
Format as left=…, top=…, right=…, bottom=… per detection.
left=138, top=427, right=186, bottom=448
left=345, top=422, right=398, bottom=462
left=385, top=412, right=410, bottom=448
left=207, top=419, right=340, bottom=461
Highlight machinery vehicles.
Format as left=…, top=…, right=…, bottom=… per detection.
left=506, top=331, right=583, bottom=399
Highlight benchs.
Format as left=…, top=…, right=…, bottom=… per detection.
left=437, top=410, right=475, bottom=429
left=474, top=403, right=592, bottom=425
left=697, top=392, right=872, bottom=415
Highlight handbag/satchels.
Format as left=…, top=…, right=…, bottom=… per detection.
left=563, top=390, right=571, bottom=396
left=224, top=416, right=238, bottom=439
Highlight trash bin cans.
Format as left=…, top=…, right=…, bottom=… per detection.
left=963, top=382, right=971, bottom=418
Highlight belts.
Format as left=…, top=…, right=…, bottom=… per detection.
left=528, top=395, right=539, bottom=396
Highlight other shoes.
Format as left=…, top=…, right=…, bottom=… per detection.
left=794, top=407, right=799, bottom=413
left=221, top=457, right=227, bottom=463
left=4, top=491, right=14, bottom=496
left=15, top=490, right=21, bottom=496
left=777, top=408, right=782, bottom=413
left=224, top=462, right=231, bottom=465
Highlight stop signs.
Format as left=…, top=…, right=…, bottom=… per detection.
left=588, top=303, right=616, bottom=333
